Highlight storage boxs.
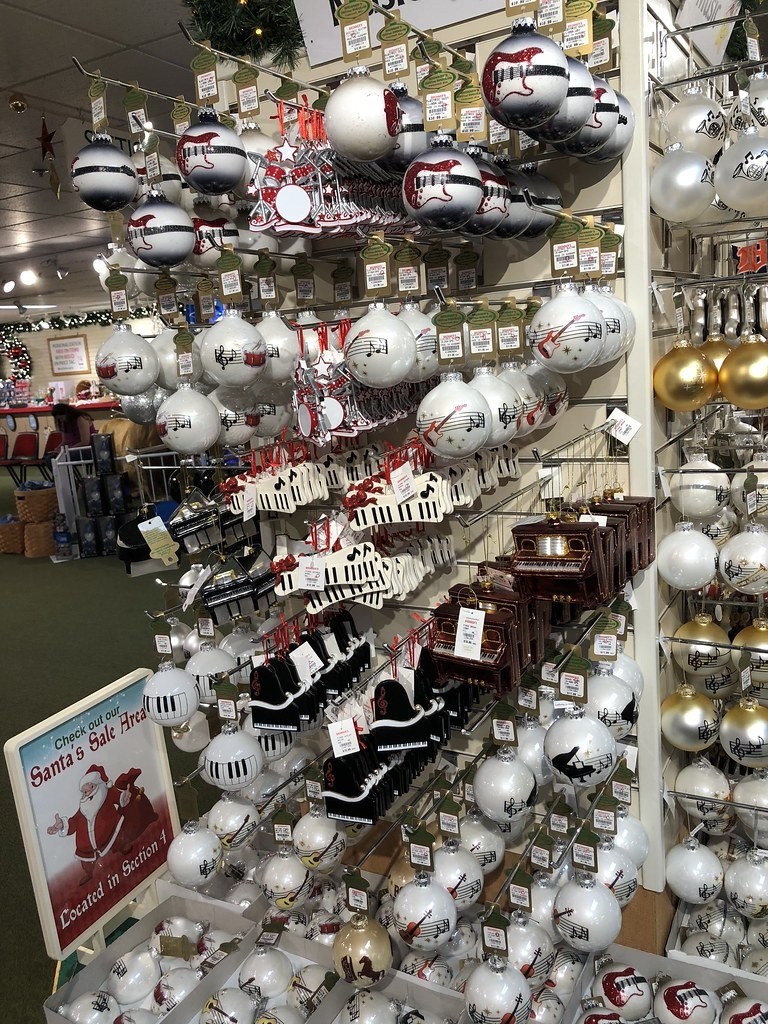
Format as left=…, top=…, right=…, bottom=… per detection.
left=44, top=625, right=768, bottom=1024
left=76, top=431, right=140, bottom=559
left=49, top=381, right=74, bottom=402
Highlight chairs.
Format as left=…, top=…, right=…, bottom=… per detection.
left=0, top=431, right=63, bottom=487
left=78, top=417, right=152, bottom=502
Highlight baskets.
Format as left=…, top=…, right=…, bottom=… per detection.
left=24, top=522, right=56, bottom=558
left=14, top=487, right=59, bottom=523
left=0, top=523, right=23, bottom=554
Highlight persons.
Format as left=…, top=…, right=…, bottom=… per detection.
left=44, top=403, right=97, bottom=482
